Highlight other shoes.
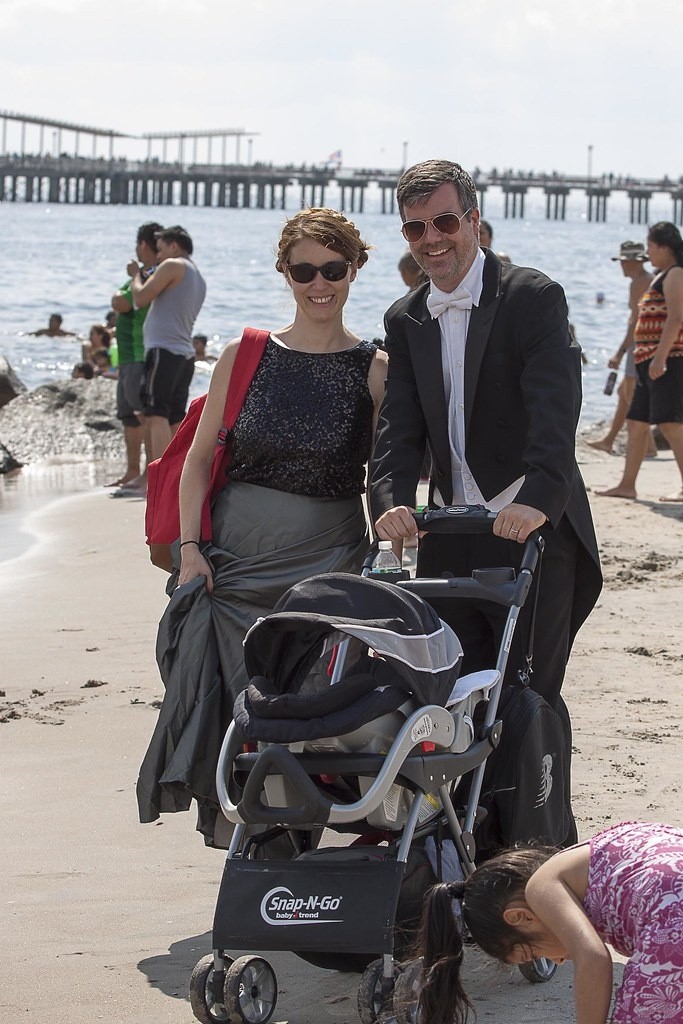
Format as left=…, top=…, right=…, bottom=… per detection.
left=110, top=488, right=145, bottom=499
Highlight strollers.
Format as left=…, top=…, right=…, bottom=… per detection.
left=188, top=503, right=546, bottom=1023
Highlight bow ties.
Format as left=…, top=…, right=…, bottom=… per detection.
left=427, top=286, right=472, bottom=319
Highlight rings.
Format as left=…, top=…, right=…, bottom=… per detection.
left=511, top=529, right=518, bottom=532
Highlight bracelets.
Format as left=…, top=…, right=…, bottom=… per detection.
left=180, top=540, right=199, bottom=547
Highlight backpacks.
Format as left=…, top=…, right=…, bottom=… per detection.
left=449, top=680, right=570, bottom=858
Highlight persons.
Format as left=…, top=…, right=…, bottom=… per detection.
left=73, top=311, right=120, bottom=378
left=594, top=221, right=683, bottom=501
left=103, top=222, right=166, bottom=489
left=585, top=241, right=656, bottom=452
left=413, top=821, right=683, bottom=1024
left=366, top=159, right=600, bottom=847
left=111, top=224, right=206, bottom=497
left=480, top=221, right=511, bottom=262
left=136, top=208, right=389, bottom=851
left=35, top=313, right=68, bottom=336
left=397, top=251, right=420, bottom=289
left=192, top=335, right=207, bottom=361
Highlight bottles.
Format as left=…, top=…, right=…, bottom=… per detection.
left=372, top=541, right=402, bottom=574
left=603, top=369, right=617, bottom=396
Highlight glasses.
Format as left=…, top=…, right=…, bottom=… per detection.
left=400, top=208, right=471, bottom=243
left=285, top=258, right=351, bottom=284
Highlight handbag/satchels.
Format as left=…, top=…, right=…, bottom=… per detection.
left=143, top=328, right=270, bottom=571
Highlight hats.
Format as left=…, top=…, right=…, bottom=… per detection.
left=610, top=241, right=649, bottom=262
